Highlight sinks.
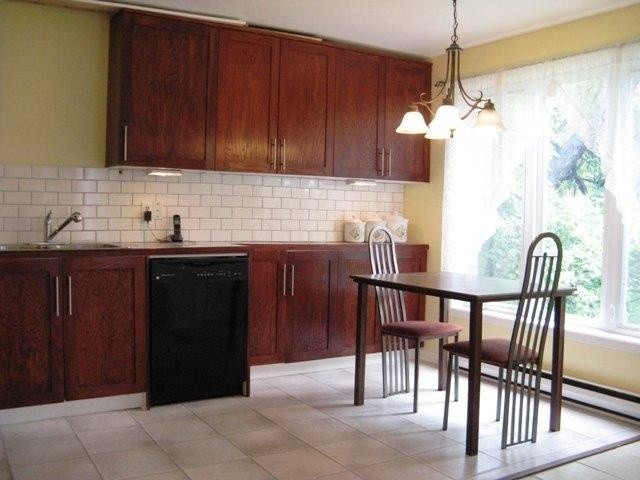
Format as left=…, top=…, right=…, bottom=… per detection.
left=57, top=244, right=118, bottom=249
left=0, top=243, right=52, bottom=250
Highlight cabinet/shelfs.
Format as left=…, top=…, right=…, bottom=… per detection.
left=103, top=8, right=213, bottom=176
left=341, top=242, right=433, bottom=357
left=209, top=18, right=332, bottom=179
left=0, top=249, right=146, bottom=409
left=245, top=244, right=342, bottom=369
left=328, top=38, right=432, bottom=187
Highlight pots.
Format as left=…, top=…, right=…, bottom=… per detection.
left=343, top=210, right=410, bottom=243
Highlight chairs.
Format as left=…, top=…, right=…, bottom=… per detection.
left=439, top=230, right=567, bottom=453
left=365, top=221, right=463, bottom=418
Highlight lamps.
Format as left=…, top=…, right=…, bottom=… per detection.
left=389, top=0, right=510, bottom=146
left=344, top=176, right=379, bottom=189
left=145, top=168, right=184, bottom=178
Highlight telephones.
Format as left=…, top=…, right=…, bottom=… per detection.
left=171, top=215, right=183, bottom=242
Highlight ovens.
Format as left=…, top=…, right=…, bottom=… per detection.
left=145, top=251, right=251, bottom=409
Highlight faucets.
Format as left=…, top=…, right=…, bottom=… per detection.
left=45, top=212, right=83, bottom=240
